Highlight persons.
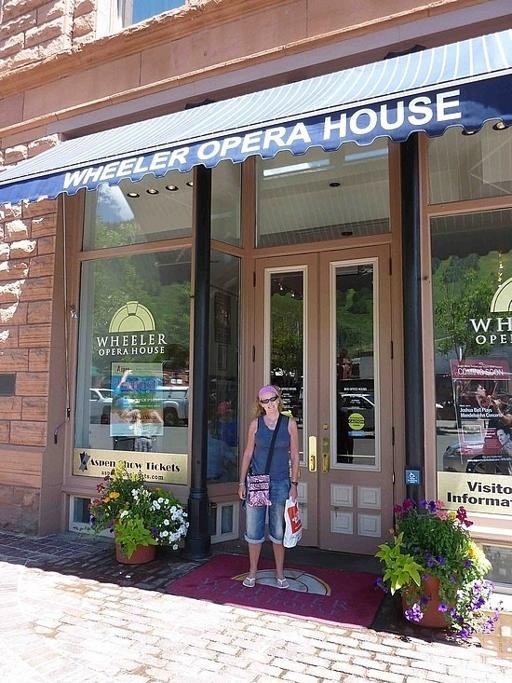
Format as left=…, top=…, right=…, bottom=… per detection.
left=460, top=384, right=492, bottom=408
left=113, top=369, right=141, bottom=422
left=205, top=397, right=239, bottom=483
left=496, top=426, right=511, bottom=474
left=483, top=394, right=512, bottom=474
left=338, top=394, right=354, bottom=463
left=237, top=385, right=300, bottom=589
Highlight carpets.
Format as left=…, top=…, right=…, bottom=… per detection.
left=166, top=552, right=388, bottom=629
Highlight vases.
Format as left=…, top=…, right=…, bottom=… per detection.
left=113, top=519, right=156, bottom=564
left=401, top=571, right=459, bottom=628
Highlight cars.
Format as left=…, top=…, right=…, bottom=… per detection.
left=443, top=440, right=504, bottom=474
left=337, top=392, right=375, bottom=429
left=89, top=384, right=189, bottom=426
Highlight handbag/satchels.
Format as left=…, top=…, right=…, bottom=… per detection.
left=247, top=473, right=272, bottom=507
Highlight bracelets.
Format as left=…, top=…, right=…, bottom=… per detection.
left=238, top=482, right=246, bottom=487
left=291, top=482, right=298, bottom=486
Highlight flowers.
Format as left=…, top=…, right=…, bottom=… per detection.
left=373, top=497, right=504, bottom=641
left=87, top=459, right=190, bottom=561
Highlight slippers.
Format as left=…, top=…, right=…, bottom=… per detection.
left=276, top=577, right=289, bottom=588
left=243, top=577, right=256, bottom=588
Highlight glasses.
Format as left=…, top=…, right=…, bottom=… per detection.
left=260, top=396, right=278, bottom=403
